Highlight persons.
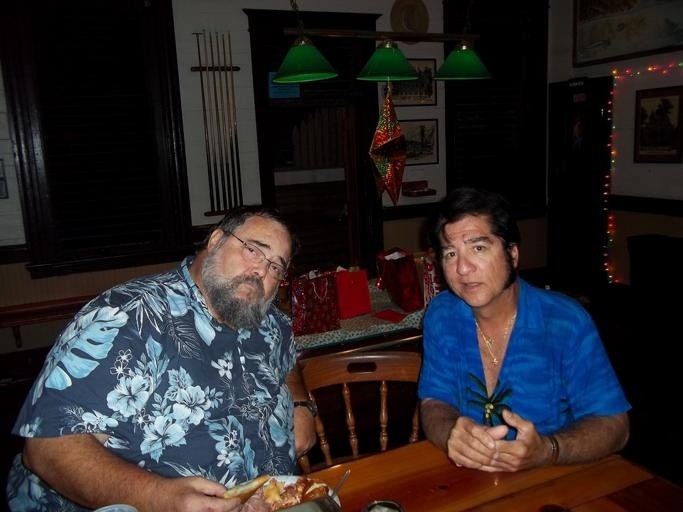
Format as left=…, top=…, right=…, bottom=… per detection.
left=6, top=203, right=321, bottom=512
left=415, top=186, right=629, bottom=473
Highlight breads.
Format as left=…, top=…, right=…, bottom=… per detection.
left=224, top=473, right=333, bottom=512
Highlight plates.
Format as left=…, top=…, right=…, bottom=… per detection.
left=242, top=475, right=342, bottom=508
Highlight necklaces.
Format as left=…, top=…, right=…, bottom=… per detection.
left=473, top=311, right=516, bottom=365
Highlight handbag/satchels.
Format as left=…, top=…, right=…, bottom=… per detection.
left=290, top=247, right=441, bottom=336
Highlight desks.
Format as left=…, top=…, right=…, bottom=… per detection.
left=295, top=277, right=425, bottom=462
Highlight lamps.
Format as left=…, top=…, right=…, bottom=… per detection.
left=272, top=0, right=493, bottom=85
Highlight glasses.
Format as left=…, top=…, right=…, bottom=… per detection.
left=227, top=230, right=289, bottom=282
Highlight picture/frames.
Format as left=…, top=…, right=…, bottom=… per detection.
left=633, top=85, right=682, bottom=164
left=384, top=58, right=439, bottom=166
left=572, top=0, right=683, bottom=68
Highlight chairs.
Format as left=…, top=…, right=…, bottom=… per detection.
left=296, top=352, right=426, bottom=474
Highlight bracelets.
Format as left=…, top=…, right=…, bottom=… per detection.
left=549, top=434, right=559, bottom=465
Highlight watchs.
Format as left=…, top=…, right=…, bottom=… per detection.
left=292, top=400, right=316, bottom=416
